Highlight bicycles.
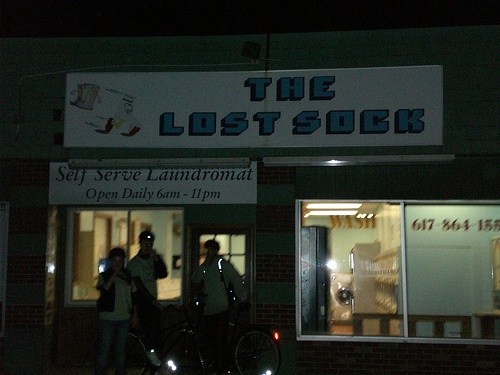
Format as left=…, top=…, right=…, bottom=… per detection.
left=125, top=291, right=282, bottom=375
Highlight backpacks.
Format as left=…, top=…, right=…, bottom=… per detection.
left=217, top=258, right=237, bottom=301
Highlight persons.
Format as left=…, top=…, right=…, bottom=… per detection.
left=191, top=240, right=240, bottom=375
left=126, top=231, right=167, bottom=367
left=93, top=248, right=138, bottom=375
